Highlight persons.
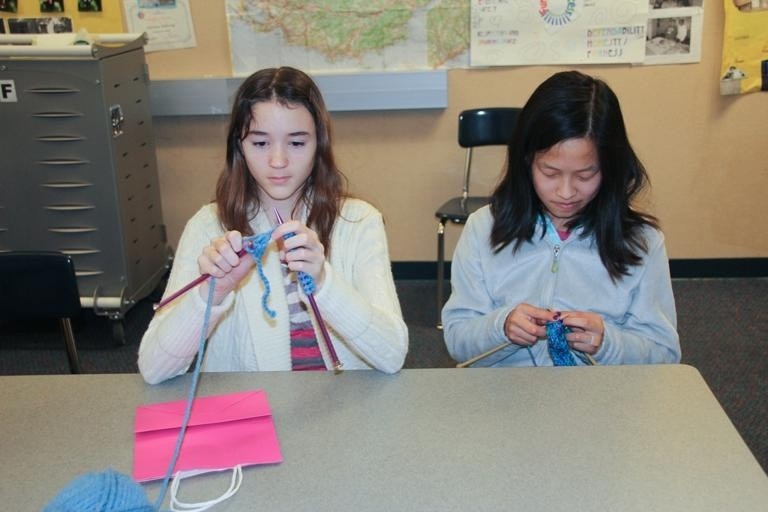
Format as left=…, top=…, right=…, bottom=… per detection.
left=441, top=69, right=685, bottom=368
left=136, top=65, right=411, bottom=386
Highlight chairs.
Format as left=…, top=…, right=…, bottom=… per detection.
left=430, top=106, right=526, bottom=331
left=0, top=251, right=87, bottom=373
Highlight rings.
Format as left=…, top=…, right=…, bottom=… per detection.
left=587, top=331, right=595, bottom=347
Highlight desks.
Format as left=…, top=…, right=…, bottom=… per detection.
left=2, top=364, right=767, bottom=509
